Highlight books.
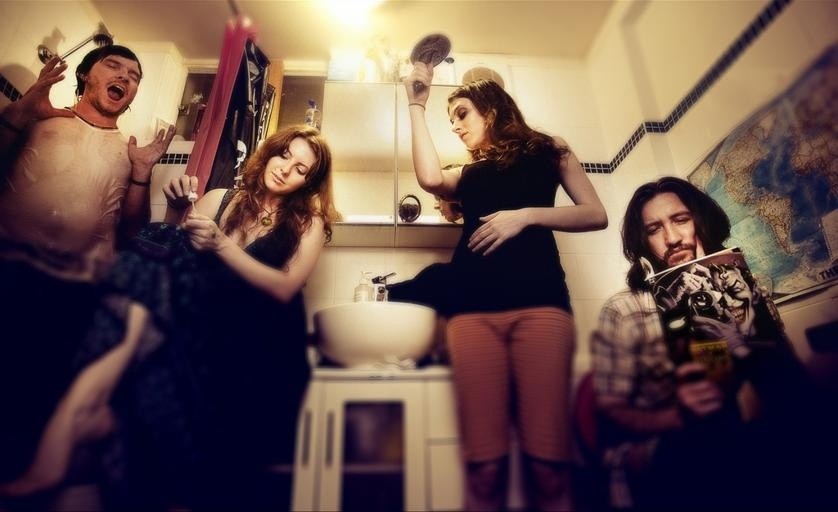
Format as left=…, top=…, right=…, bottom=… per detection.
left=637, top=247, right=781, bottom=387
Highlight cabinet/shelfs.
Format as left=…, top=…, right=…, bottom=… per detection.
left=291, top=367, right=466, bottom=511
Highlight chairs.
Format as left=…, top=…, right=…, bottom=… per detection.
left=575, top=375, right=601, bottom=452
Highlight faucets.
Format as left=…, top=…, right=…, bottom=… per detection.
left=372, top=272, right=396, bottom=301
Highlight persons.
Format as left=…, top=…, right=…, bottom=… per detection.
left=404, top=60, right=609, bottom=511
left=592, top=176, right=838, bottom=512
left=654, top=264, right=779, bottom=369
left=2, top=44, right=339, bottom=511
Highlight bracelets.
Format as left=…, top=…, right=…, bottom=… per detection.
left=408, top=102, right=426, bottom=111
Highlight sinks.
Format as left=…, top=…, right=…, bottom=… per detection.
left=313, top=302, right=437, bottom=368
